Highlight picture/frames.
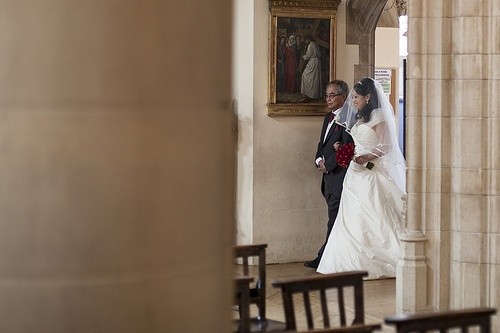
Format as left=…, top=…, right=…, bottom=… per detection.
left=266, top=6, right=339, bottom=117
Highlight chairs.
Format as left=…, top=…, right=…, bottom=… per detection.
left=272, top=269, right=369, bottom=333
left=233, top=244, right=268, bottom=321
left=382, top=307, right=497, bottom=333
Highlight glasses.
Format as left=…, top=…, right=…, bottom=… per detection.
left=323, top=94, right=342, bottom=99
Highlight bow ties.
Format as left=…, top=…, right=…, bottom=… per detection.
left=327, top=113, right=335, bottom=123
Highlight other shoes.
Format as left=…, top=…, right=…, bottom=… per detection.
left=305, top=256, right=322, bottom=268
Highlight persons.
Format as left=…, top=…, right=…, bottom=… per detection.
left=303, top=79, right=357, bottom=268
left=333, top=78, right=401, bottom=275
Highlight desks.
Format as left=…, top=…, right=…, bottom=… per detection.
left=233, top=317, right=296, bottom=333
left=234, top=276, right=254, bottom=321
